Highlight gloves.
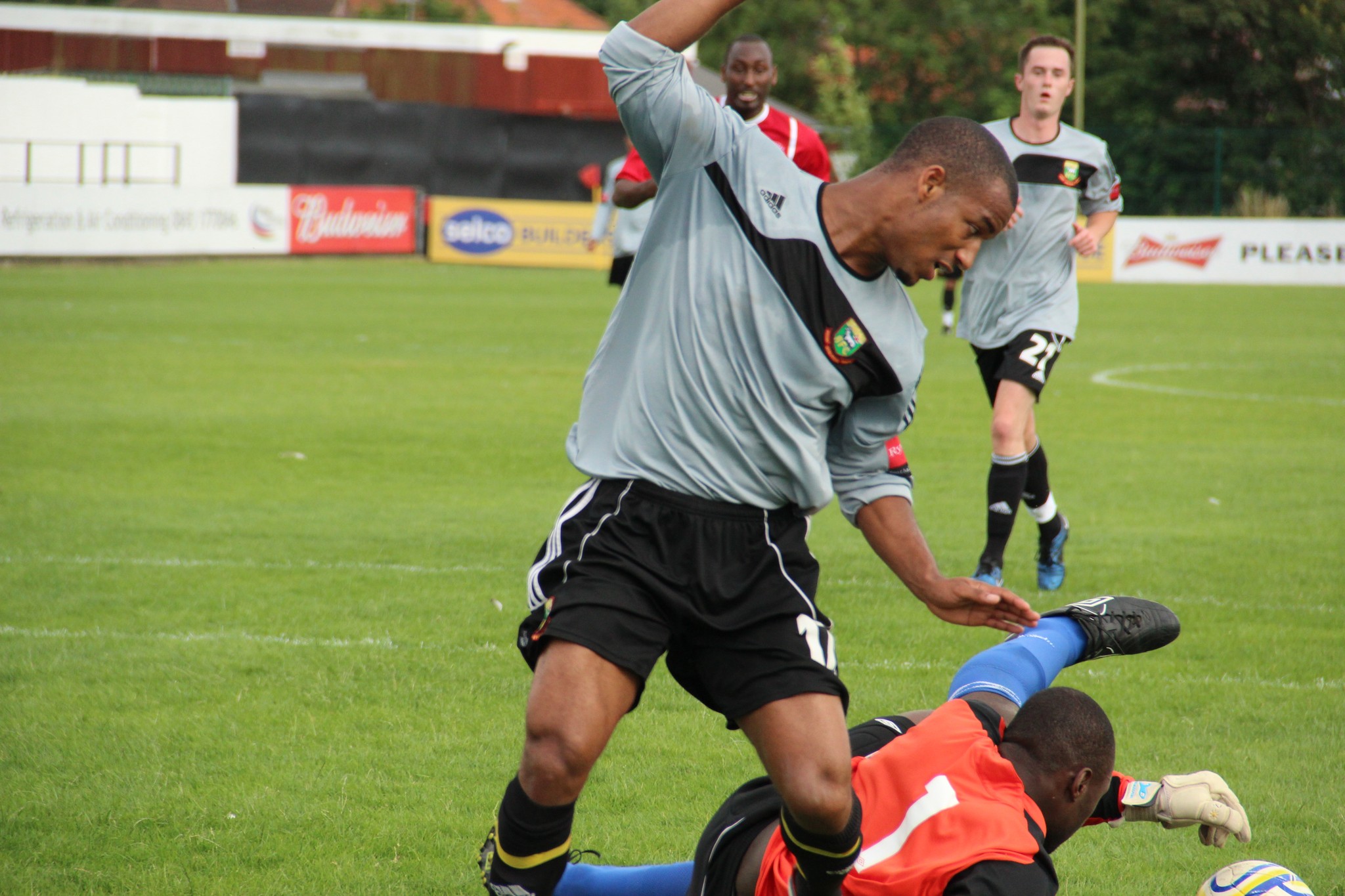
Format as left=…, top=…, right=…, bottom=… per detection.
left=1119, top=768, right=1251, bottom=850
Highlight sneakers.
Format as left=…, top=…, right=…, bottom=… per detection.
left=1037, top=516, right=1069, bottom=591
left=1039, top=596, right=1180, bottom=668
left=969, top=562, right=1005, bottom=589
left=477, top=823, right=601, bottom=893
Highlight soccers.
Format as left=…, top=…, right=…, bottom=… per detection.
left=1196, top=861, right=1317, bottom=896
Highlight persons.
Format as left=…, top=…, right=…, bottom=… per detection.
left=485, top=0, right=1042, bottom=896
left=477, top=596, right=1251, bottom=896
left=936, top=35, right=1122, bottom=591
left=612, top=34, right=831, bottom=208
left=588, top=133, right=653, bottom=286
